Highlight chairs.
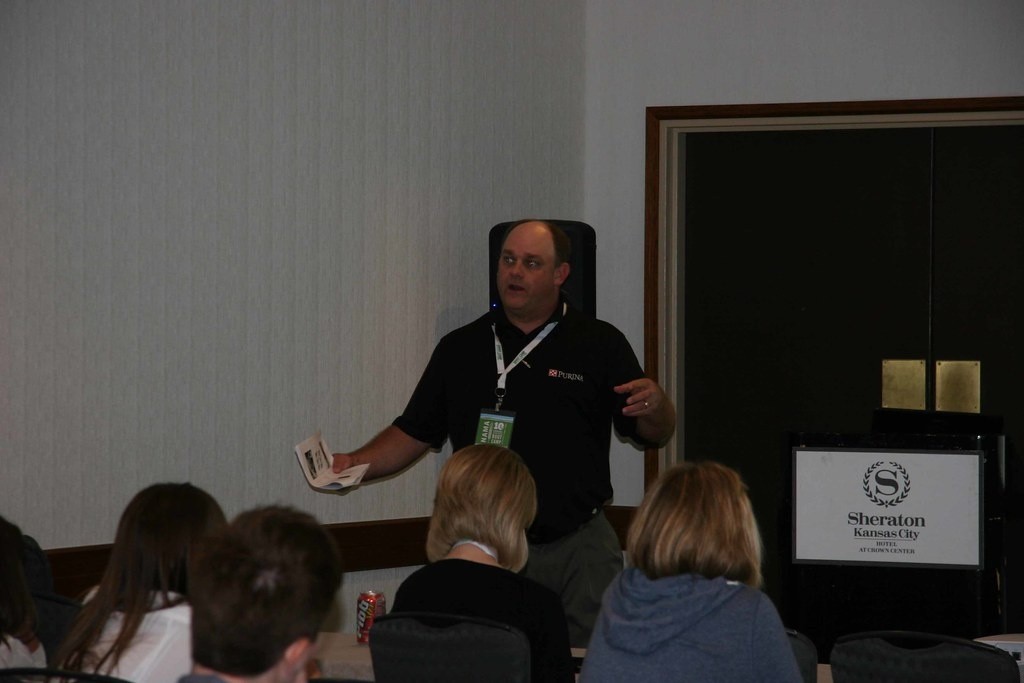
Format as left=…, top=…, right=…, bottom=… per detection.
left=370, top=611, right=1020, bottom=683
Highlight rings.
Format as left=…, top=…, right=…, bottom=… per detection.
left=643, top=399, right=648, bottom=408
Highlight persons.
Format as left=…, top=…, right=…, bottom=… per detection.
left=332, top=220, right=679, bottom=649
left=0, top=484, right=344, bottom=683
left=389, top=442, right=577, bottom=683
left=577, top=459, right=803, bottom=683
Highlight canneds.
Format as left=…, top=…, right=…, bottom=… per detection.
left=356, top=591, right=386, bottom=645
